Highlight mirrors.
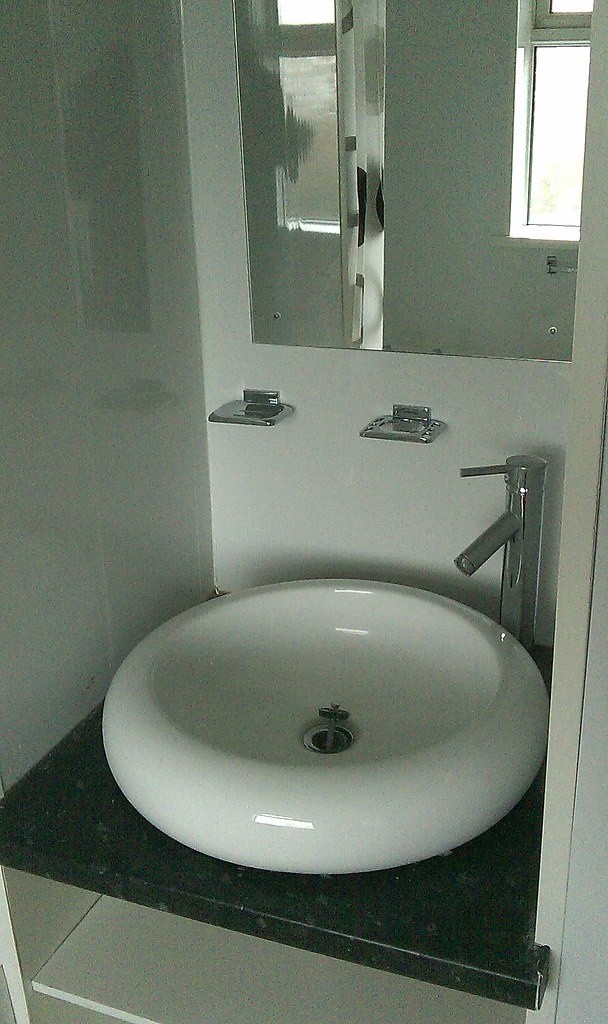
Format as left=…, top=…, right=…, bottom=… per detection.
left=231, top=0, right=595, bottom=363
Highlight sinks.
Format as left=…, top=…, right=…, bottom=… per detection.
left=102, top=578, right=549, bottom=875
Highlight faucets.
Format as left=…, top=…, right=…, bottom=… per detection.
left=453, top=455, right=547, bottom=648
